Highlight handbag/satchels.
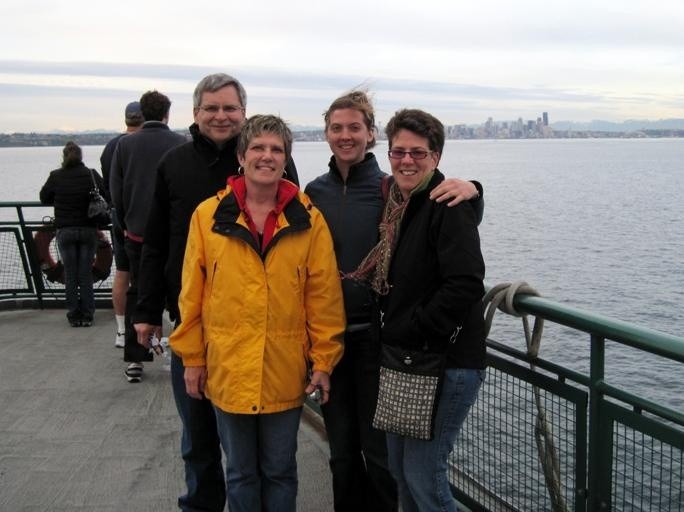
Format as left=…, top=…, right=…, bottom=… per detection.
left=87, top=187, right=114, bottom=225
left=372, top=344, right=447, bottom=442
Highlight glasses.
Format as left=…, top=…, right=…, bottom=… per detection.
left=388, top=148, right=434, bottom=161
left=195, top=104, right=244, bottom=115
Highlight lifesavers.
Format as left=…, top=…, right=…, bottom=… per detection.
left=33, top=219, right=113, bottom=284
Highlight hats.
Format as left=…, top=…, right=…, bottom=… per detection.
left=125, top=101, right=142, bottom=118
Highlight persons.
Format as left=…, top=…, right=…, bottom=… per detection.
left=97, top=100, right=145, bottom=350
left=108, top=89, right=187, bottom=385
left=301, top=89, right=488, bottom=511
left=37, top=139, right=110, bottom=327
left=166, top=113, right=347, bottom=511
left=368, top=105, right=488, bottom=511
left=129, top=73, right=301, bottom=512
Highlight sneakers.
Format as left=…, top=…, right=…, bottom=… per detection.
left=114, top=331, right=126, bottom=348
left=71, top=320, right=92, bottom=327
left=125, top=363, right=143, bottom=383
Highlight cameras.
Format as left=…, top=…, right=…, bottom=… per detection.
left=309, top=388, right=321, bottom=404
